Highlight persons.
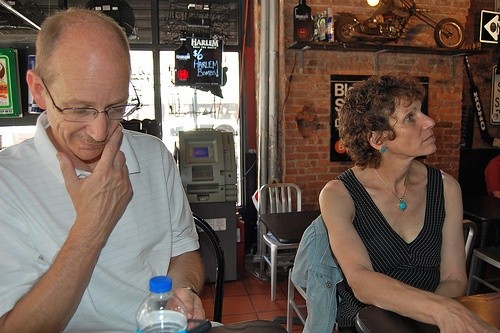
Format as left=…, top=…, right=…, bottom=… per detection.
left=0, top=9, right=205, bottom=333
left=318, top=74, right=500, bottom=333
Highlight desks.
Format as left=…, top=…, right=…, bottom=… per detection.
left=353, top=292, right=500, bottom=333
left=260, top=211, right=320, bottom=243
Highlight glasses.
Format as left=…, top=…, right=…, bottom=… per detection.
left=40, top=77, right=141, bottom=122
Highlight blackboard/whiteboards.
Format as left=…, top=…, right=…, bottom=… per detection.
left=330, top=73, right=429, bottom=163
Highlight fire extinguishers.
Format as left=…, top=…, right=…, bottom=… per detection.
left=236, top=210, right=246, bottom=280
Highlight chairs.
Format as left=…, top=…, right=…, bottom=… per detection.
left=257, top=183, right=478, bottom=333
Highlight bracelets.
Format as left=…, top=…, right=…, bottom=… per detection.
left=181, top=286, right=197, bottom=293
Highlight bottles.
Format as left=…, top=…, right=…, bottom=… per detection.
left=293, top=0, right=313, bottom=42
left=137, top=276, right=188, bottom=333
left=313, top=8, right=335, bottom=43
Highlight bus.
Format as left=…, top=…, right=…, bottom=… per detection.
left=162, top=100, right=239, bottom=138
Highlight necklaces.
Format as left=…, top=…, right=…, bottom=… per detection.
left=372, top=167, right=410, bottom=212
